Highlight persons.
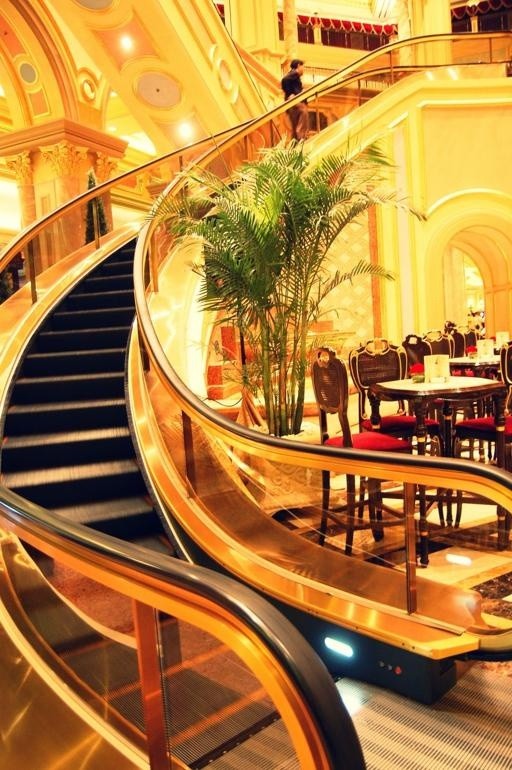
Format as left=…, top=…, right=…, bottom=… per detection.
left=282, top=60, right=309, bottom=145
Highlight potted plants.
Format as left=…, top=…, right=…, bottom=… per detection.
left=136, top=122, right=427, bottom=513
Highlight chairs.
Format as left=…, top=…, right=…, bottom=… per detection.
left=310, top=319, right=511, bottom=569
